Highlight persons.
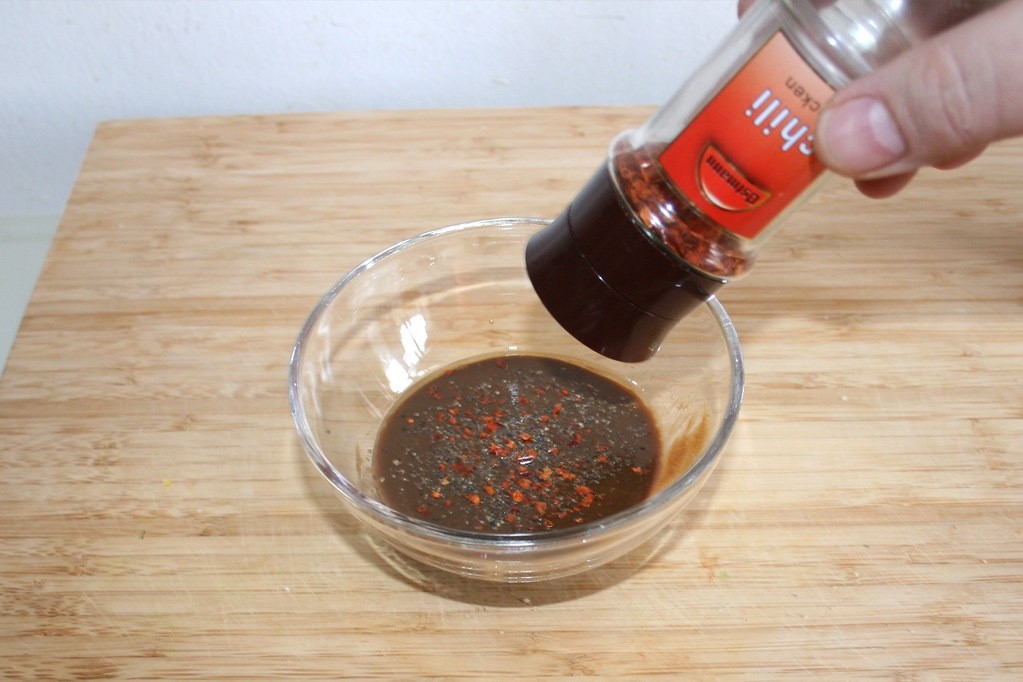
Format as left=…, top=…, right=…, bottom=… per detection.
left=738, top=0, right=1023, bottom=199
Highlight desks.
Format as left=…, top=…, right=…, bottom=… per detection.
left=1, top=107, right=1023, bottom=677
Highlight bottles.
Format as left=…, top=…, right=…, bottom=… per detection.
left=525, top=0, right=945, bottom=364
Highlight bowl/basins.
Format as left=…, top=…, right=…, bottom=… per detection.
left=287, top=217, right=746, bottom=584
